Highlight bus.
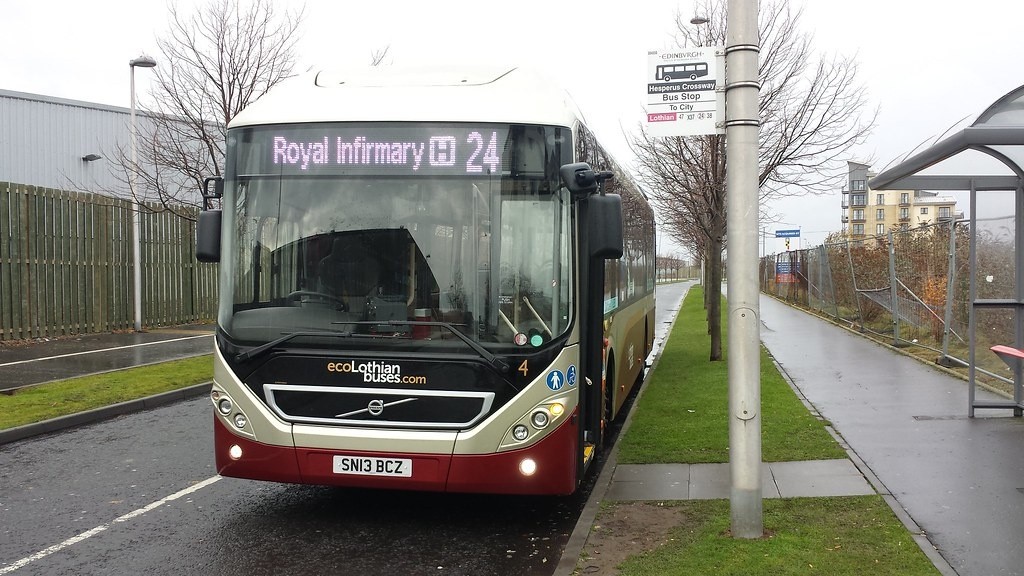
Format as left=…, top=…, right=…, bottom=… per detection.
left=193, top=65, right=656, bottom=494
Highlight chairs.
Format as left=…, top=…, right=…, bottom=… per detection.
left=319, top=247, right=391, bottom=319
left=427, top=249, right=568, bottom=324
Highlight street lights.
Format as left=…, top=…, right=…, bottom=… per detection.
left=130, top=55, right=157, bottom=332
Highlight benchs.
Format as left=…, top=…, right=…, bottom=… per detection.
left=989, top=344, right=1024, bottom=370
